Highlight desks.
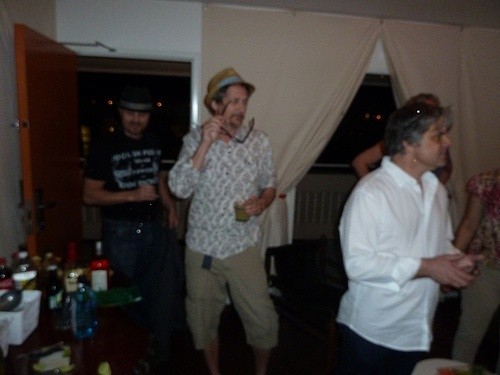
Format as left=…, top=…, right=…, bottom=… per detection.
left=0, top=305, right=153, bottom=375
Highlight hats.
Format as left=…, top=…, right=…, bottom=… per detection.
left=204, top=67, right=255, bottom=116
left=116, top=74, right=156, bottom=112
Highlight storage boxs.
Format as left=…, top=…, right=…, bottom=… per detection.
left=0, top=289, right=43, bottom=346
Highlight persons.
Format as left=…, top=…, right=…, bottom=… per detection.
left=439, top=169, right=500, bottom=366
left=351, top=94, right=451, bottom=182
left=334, top=105, right=486, bottom=375
left=169, top=66, right=280, bottom=375
left=83, top=91, right=181, bottom=364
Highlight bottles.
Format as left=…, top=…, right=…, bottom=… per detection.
left=0, top=242, right=108, bottom=339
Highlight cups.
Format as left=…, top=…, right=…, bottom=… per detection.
left=234, top=202, right=251, bottom=222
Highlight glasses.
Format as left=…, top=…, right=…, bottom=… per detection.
left=220, top=99, right=253, bottom=143
